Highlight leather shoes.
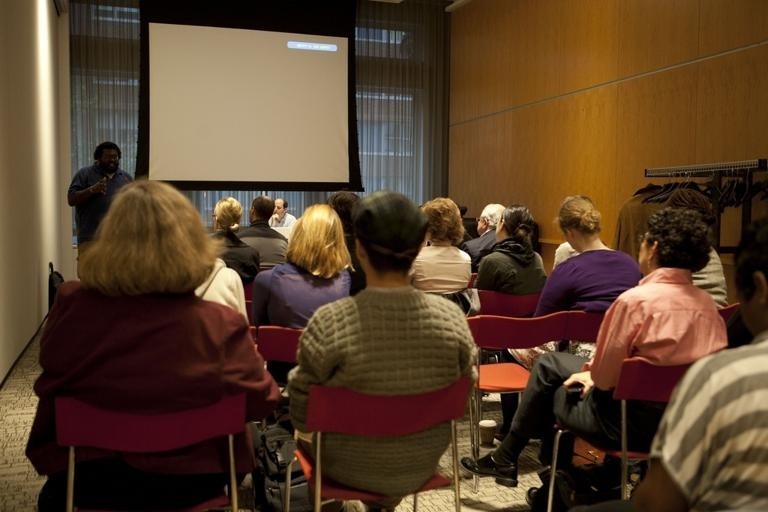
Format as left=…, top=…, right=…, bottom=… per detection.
left=525, top=487, right=545, bottom=510
left=462, top=454, right=519, bottom=487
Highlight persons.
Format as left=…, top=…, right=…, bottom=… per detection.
left=459, top=208, right=730, bottom=509
left=250, top=205, right=352, bottom=393
left=287, top=190, right=476, bottom=512
left=660, top=190, right=728, bottom=317
left=66, top=142, right=138, bottom=258
left=532, top=195, right=640, bottom=364
left=232, top=197, right=287, bottom=279
left=274, top=200, right=295, bottom=227
left=415, top=196, right=472, bottom=298
left=578, top=225, right=768, bottom=511
left=329, top=193, right=353, bottom=245
left=475, top=207, right=548, bottom=438
left=465, top=204, right=503, bottom=285
left=19, top=178, right=281, bottom=511
left=190, top=196, right=257, bottom=279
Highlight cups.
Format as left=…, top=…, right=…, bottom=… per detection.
left=480, top=421, right=497, bottom=444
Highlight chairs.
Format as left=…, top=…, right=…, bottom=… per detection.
left=477, top=288, right=543, bottom=318
left=570, top=308, right=604, bottom=342
left=468, top=271, right=478, bottom=288
left=720, top=300, right=743, bottom=324
left=243, top=282, right=254, bottom=326
left=54, top=389, right=249, bottom=511
left=465, top=310, right=579, bottom=465
left=286, top=373, right=470, bottom=512
left=545, top=356, right=689, bottom=511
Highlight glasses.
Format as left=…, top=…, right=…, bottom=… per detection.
left=475, top=216, right=484, bottom=223
left=637, top=233, right=654, bottom=242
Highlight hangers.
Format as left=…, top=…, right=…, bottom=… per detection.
left=641, top=159, right=758, bottom=205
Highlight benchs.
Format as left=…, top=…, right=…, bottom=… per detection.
left=222, top=323, right=303, bottom=385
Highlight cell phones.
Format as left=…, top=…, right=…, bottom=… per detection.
left=567, top=385, right=582, bottom=393
